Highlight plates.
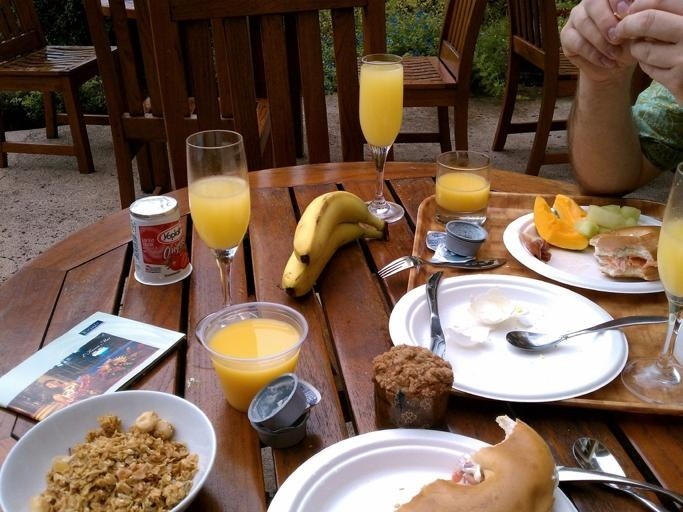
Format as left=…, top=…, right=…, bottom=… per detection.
left=262, top=428, right=581, bottom=512
left=388, top=273, right=630, bottom=404
left=503, top=205, right=666, bottom=294
left=0, top=391, right=217, bottom=512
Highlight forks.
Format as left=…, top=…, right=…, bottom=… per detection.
left=378, top=256, right=506, bottom=279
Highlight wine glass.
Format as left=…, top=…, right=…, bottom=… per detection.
left=356, top=54, right=405, bottom=225
left=185, top=131, right=252, bottom=307
left=619, top=163, right=682, bottom=406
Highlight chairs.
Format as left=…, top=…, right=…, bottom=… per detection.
left=357, top=1, right=486, bottom=168
left=0, top=1, right=118, bottom=176
left=488, top=1, right=582, bottom=176
left=82, top=1, right=271, bottom=211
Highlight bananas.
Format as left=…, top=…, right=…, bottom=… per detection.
left=281, top=190, right=389, bottom=300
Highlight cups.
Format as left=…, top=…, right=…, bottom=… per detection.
left=436, top=148, right=488, bottom=229
left=195, top=301, right=310, bottom=447
left=445, top=218, right=485, bottom=258
left=127, top=196, right=193, bottom=288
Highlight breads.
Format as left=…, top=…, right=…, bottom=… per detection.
left=590, top=226, right=662, bottom=281
left=394, top=415, right=559, bottom=512
left=370, top=344, right=453, bottom=429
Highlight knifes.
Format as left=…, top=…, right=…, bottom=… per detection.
left=423, top=269, right=447, bottom=363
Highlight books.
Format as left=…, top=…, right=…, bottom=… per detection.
left=0, top=311, right=187, bottom=422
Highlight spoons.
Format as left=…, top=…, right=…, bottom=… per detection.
left=505, top=316, right=667, bottom=352
left=572, top=438, right=668, bottom=512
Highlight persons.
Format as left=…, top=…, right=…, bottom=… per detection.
left=560, top=0, right=683, bottom=198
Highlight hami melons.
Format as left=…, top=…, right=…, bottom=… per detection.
left=531, top=194, right=589, bottom=251
